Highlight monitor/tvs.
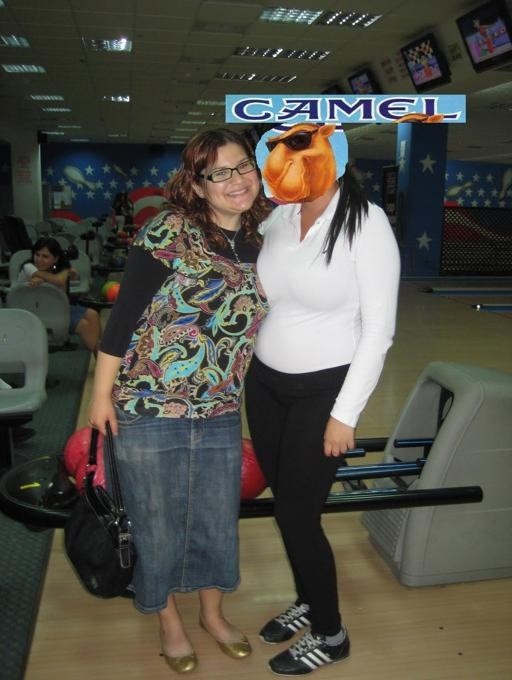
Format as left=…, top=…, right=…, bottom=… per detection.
left=454, top=0, right=512, bottom=73
left=237, top=126, right=262, bottom=155
left=321, top=85, right=347, bottom=94
left=347, top=68, right=383, bottom=94
left=399, top=32, right=452, bottom=94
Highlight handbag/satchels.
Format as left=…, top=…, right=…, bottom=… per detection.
left=62, top=418, right=139, bottom=600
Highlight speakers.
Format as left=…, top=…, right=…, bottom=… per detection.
left=37, top=133, right=48, bottom=144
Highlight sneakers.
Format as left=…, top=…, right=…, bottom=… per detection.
left=266, top=623, right=352, bottom=678
left=258, top=596, right=314, bottom=646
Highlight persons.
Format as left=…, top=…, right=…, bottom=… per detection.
left=16, top=237, right=101, bottom=359
left=124, top=192, right=134, bottom=225
left=111, top=192, right=128, bottom=232
left=245, top=124, right=402, bottom=677
left=84, top=128, right=269, bottom=674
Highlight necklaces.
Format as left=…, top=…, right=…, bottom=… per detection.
left=219, top=227, right=240, bottom=262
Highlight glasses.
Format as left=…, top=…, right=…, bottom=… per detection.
left=198, top=159, right=257, bottom=184
left=265, top=130, right=321, bottom=153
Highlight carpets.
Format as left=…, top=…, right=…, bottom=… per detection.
left=2, top=298, right=102, bottom=680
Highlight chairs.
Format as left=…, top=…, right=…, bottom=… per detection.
left=1, top=207, right=129, bottom=474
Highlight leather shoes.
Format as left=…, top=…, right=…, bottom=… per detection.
left=158, top=629, right=199, bottom=675
left=197, top=612, right=254, bottom=660
